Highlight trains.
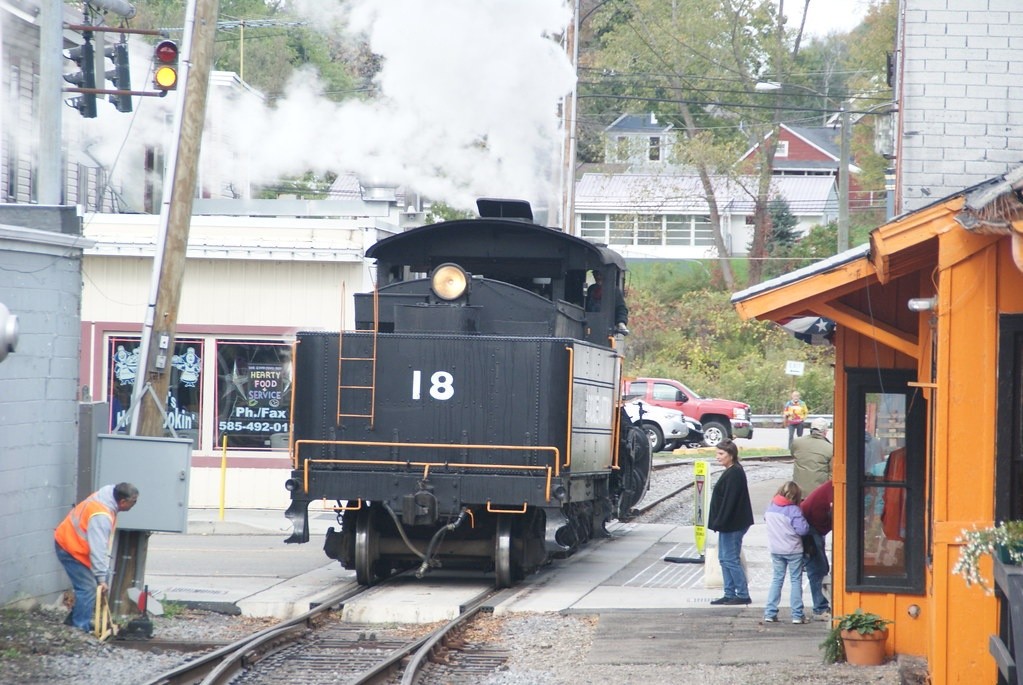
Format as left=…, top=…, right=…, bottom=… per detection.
left=285, top=200, right=651, bottom=588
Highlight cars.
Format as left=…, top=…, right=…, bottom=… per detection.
left=620, top=376, right=754, bottom=449
left=625, top=402, right=688, bottom=454
left=663, top=416, right=704, bottom=453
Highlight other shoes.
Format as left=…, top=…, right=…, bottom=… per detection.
left=793, top=617, right=810, bottom=623
left=814, top=612, right=830, bottom=621
left=765, top=617, right=778, bottom=621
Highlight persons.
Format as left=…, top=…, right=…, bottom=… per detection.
left=788, top=418, right=833, bottom=574
left=763, top=412, right=888, bottom=625
left=799, top=472, right=876, bottom=622
left=863, top=415, right=886, bottom=472
left=764, top=480, right=811, bottom=623
left=708, top=438, right=753, bottom=605
left=784, top=391, right=808, bottom=452
left=54, top=482, right=139, bottom=633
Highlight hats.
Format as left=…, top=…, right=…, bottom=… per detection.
left=810, top=417, right=828, bottom=431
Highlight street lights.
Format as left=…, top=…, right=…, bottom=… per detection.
left=755, top=81, right=850, bottom=253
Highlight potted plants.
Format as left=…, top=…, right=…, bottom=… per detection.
left=822, top=609, right=890, bottom=667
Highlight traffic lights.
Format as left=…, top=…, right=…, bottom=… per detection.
left=153, top=38, right=180, bottom=90
left=63, top=44, right=97, bottom=119
left=104, top=42, right=133, bottom=113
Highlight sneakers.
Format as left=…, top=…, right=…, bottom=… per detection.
left=711, top=597, right=730, bottom=604
left=723, top=596, right=751, bottom=604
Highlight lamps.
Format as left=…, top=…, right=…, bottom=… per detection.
left=908, top=296, right=939, bottom=313
